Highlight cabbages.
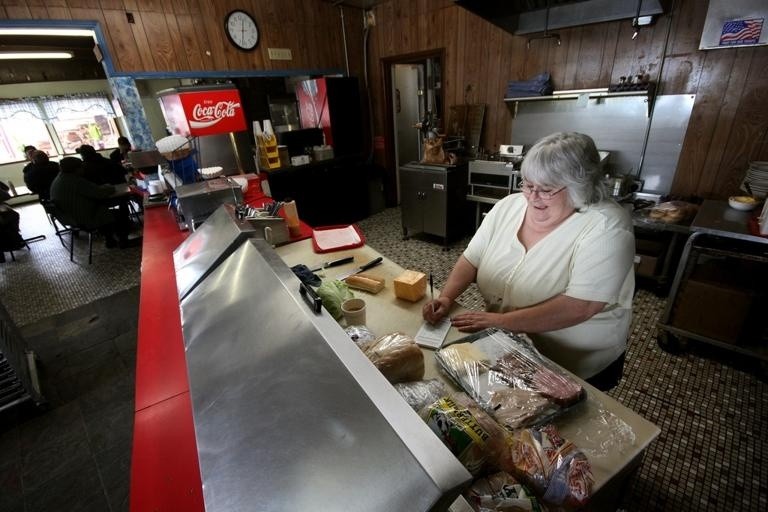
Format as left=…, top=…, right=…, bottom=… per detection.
left=315, top=280, right=354, bottom=320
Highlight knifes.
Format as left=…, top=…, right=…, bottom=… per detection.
left=336, top=256, right=383, bottom=282
left=310, top=255, right=356, bottom=272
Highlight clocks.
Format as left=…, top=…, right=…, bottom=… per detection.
left=224, top=9, right=259, bottom=50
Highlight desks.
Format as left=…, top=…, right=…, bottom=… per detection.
left=273, top=234, right=661, bottom=512
left=656, top=200, right=768, bottom=366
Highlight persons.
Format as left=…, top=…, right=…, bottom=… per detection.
left=25, top=150, right=61, bottom=195
left=21, top=144, right=38, bottom=173
left=109, top=136, right=131, bottom=159
left=75, top=144, right=131, bottom=216
left=46, top=156, right=142, bottom=250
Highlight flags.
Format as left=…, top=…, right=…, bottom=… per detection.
left=719, top=18, right=763, bottom=46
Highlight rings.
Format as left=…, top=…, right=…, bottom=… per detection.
left=469, top=319, right=472, bottom=325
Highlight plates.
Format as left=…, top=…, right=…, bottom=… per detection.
left=740, top=160, right=768, bottom=200
left=304, top=145, right=335, bottom=161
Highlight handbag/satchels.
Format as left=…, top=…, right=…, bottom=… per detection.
left=252, top=119, right=281, bottom=169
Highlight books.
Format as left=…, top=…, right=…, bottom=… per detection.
left=415, top=315, right=454, bottom=350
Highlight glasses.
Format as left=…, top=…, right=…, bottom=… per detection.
left=517, top=180, right=567, bottom=201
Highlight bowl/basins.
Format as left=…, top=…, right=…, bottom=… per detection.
left=728, top=195, right=758, bottom=212
left=198, top=166, right=224, bottom=180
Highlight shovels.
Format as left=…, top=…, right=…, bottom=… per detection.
left=308, top=255, right=382, bottom=281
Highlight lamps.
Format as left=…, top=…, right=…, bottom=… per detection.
left=0, top=51, right=74, bottom=60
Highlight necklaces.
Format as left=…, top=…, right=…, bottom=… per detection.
left=420, top=128, right=636, bottom=392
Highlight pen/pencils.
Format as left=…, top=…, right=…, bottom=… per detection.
left=430, top=273, right=435, bottom=314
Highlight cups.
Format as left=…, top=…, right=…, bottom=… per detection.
left=148, top=185, right=158, bottom=196
left=340, top=298, right=366, bottom=328
left=252, top=119, right=281, bottom=168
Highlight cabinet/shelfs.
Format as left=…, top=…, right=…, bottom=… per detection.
left=399, top=162, right=464, bottom=247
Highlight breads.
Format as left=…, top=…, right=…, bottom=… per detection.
left=345, top=274, right=385, bottom=294
left=362, top=330, right=425, bottom=384
left=393, top=269, right=427, bottom=302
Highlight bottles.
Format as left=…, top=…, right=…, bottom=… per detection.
left=284, top=198, right=301, bottom=237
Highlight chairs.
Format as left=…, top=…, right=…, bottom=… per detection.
left=109, top=201, right=143, bottom=229
left=40, top=199, right=115, bottom=264
left=50, top=212, right=72, bottom=246
